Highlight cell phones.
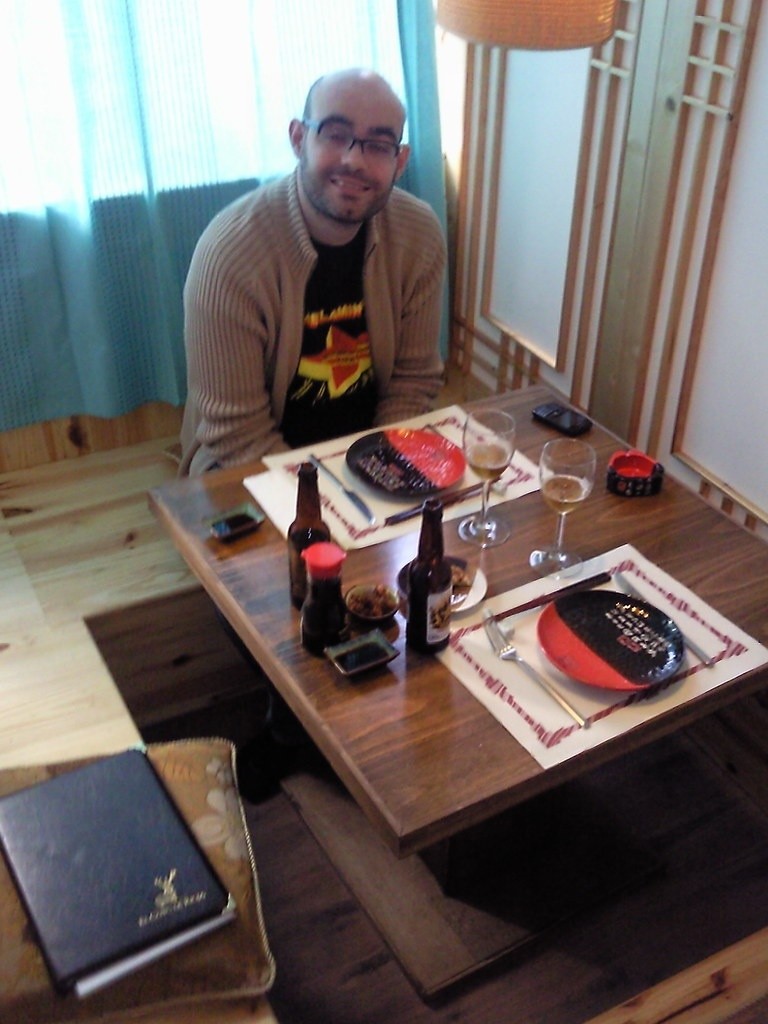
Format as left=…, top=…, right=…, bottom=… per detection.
left=531, top=402, right=592, bottom=437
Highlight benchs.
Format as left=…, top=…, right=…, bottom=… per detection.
left=0, top=363, right=768, bottom=1024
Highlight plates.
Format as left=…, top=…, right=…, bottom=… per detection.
left=537, top=589, right=684, bottom=691
left=344, top=583, right=399, bottom=623
left=397, top=556, right=488, bottom=613
left=347, top=428, right=465, bottom=498
left=200, top=502, right=267, bottom=543
left=324, top=628, right=401, bottom=676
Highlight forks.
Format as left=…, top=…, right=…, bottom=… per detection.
left=481, top=617, right=587, bottom=727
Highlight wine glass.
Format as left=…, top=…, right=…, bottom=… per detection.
left=459, top=408, right=516, bottom=546
left=529, top=439, right=597, bottom=578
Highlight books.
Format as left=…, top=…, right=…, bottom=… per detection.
left=0, top=749, right=240, bottom=1004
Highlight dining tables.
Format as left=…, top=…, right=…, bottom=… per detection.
left=145, top=387, right=768, bottom=999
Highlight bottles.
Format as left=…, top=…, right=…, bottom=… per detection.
left=301, top=544, right=351, bottom=660
left=405, top=499, right=452, bottom=655
left=290, top=462, right=330, bottom=613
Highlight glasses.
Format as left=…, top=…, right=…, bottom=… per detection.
left=302, top=115, right=403, bottom=159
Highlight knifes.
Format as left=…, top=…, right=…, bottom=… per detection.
left=309, top=454, right=373, bottom=522
left=613, top=573, right=715, bottom=667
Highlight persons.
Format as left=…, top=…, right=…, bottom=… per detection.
left=176, top=69, right=446, bottom=481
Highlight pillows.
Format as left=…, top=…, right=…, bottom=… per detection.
left=0, top=734, right=276, bottom=1024
